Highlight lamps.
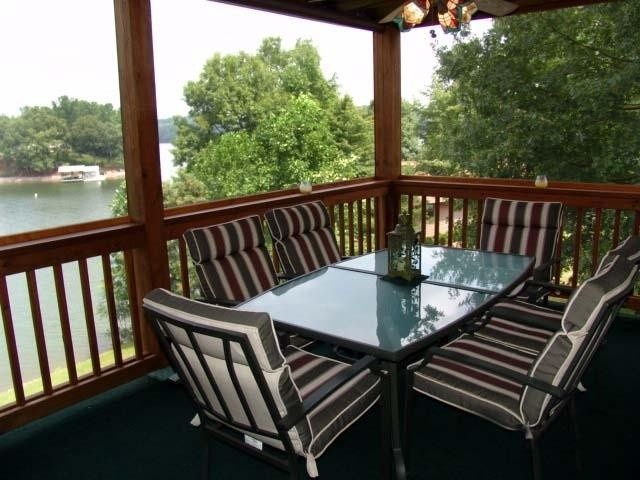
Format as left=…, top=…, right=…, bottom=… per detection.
left=393, top=0, right=477, bottom=34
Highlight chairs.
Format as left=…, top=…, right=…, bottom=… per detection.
left=263, top=199, right=340, bottom=279
left=478, top=197, right=562, bottom=305
left=141, top=287, right=408, bottom=480
left=183, top=214, right=303, bottom=351
left=410, top=257, right=632, bottom=450
left=469, top=233, right=639, bottom=403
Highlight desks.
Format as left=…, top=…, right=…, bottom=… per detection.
left=233, top=241, right=537, bottom=480
